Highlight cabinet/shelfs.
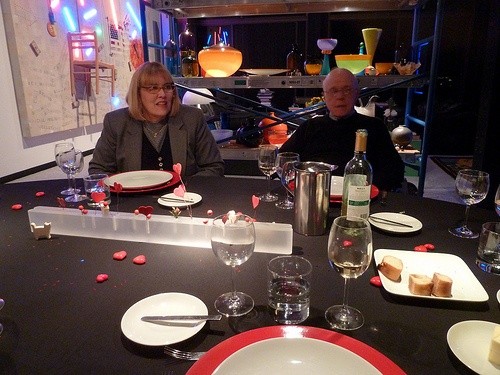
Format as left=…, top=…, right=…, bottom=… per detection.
left=139, top=0, right=437, bottom=197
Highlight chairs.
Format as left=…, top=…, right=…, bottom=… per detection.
left=68, top=31, right=115, bottom=96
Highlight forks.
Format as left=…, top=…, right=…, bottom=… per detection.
left=161, top=346, right=206, bottom=361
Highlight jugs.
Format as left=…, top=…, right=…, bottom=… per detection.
left=280, top=161, right=338, bottom=236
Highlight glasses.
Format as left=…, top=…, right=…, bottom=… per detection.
left=320, top=85, right=357, bottom=97
left=139, top=83, right=173, bottom=94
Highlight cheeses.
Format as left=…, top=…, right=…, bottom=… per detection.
left=488, top=325, right=500, bottom=366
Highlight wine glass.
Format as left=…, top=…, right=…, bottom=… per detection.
left=447, top=169, right=489, bottom=239
left=209, top=214, right=255, bottom=316
left=274, top=153, right=300, bottom=209
left=257, top=144, right=280, bottom=202
left=54, top=142, right=87, bottom=203
left=324, top=215, right=373, bottom=331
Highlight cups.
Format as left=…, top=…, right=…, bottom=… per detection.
left=82, top=174, right=111, bottom=208
left=267, top=255, right=312, bottom=325
left=494, top=186, right=500, bottom=217
left=475, top=222, right=500, bottom=275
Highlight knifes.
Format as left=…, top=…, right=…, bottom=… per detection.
left=153, top=195, right=194, bottom=203
left=141, top=315, right=222, bottom=320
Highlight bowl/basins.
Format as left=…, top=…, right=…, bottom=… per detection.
left=335, top=55, right=373, bottom=75
left=199, top=47, right=243, bottom=76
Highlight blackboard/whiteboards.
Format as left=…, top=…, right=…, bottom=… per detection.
left=0, top=0, right=174, bottom=149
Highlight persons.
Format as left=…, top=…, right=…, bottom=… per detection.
left=279, top=68, right=405, bottom=191
left=88, top=61, right=225, bottom=177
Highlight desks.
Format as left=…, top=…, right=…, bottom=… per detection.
left=0, top=177, right=500, bottom=375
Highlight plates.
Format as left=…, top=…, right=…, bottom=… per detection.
left=239, top=68, right=291, bottom=75
left=121, top=292, right=209, bottom=346
left=287, top=176, right=379, bottom=203
left=446, top=320, right=500, bottom=375
left=97, top=170, right=180, bottom=193
left=368, top=212, right=422, bottom=232
left=158, top=192, right=202, bottom=207
left=374, top=248, right=489, bottom=302
left=186, top=325, right=407, bottom=375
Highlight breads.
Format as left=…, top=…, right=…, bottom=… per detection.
left=409, top=272, right=453, bottom=297
left=377, top=255, right=403, bottom=280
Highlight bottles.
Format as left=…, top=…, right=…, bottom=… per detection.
left=341, top=129, right=373, bottom=236
left=391, top=124, right=413, bottom=145
